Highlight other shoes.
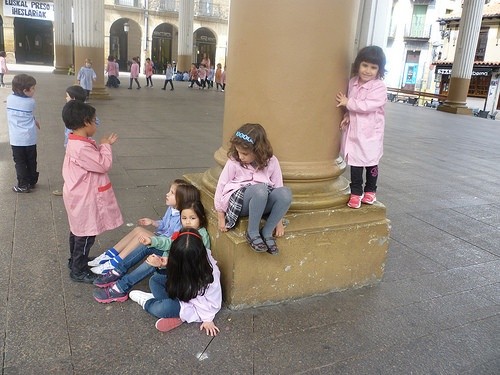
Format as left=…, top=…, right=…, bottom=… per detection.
left=136, top=86, right=141, bottom=89
left=170, top=89, right=174, bottom=91
left=197, top=86, right=201, bottom=90
left=127, top=88, right=132, bottom=89
left=29, top=184, right=36, bottom=189
left=161, top=88, right=166, bottom=90
left=53, top=190, right=62, bottom=195
left=187, top=86, right=193, bottom=88
left=146, top=84, right=153, bottom=87
left=203, top=87, right=226, bottom=91
left=260, top=228, right=278, bottom=254
left=245, top=232, right=268, bottom=252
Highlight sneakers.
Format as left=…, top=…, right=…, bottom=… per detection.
left=361, top=192, right=376, bottom=204
left=88, top=251, right=106, bottom=266
left=347, top=194, right=362, bottom=209
left=129, top=289, right=155, bottom=309
left=69, top=257, right=95, bottom=269
left=92, top=269, right=120, bottom=288
left=91, top=262, right=114, bottom=275
left=93, top=283, right=128, bottom=303
left=155, top=318, right=182, bottom=332
left=12, top=185, right=31, bottom=193
left=72, top=271, right=101, bottom=283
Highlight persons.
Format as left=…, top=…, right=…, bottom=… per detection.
left=105, top=55, right=120, bottom=88
left=336, top=46, right=389, bottom=208
left=5, top=73, right=40, bottom=194
left=212, top=123, right=292, bottom=256
left=77, top=57, right=97, bottom=100
left=53, top=85, right=100, bottom=195
left=62, top=99, right=125, bottom=284
left=162, top=62, right=175, bottom=91
left=174, top=52, right=226, bottom=92
left=145, top=58, right=153, bottom=88
left=89, top=179, right=222, bottom=337
left=0, top=50, right=8, bottom=88
left=128, top=57, right=141, bottom=90
left=68, top=64, right=76, bottom=75
left=115, top=58, right=176, bottom=74
